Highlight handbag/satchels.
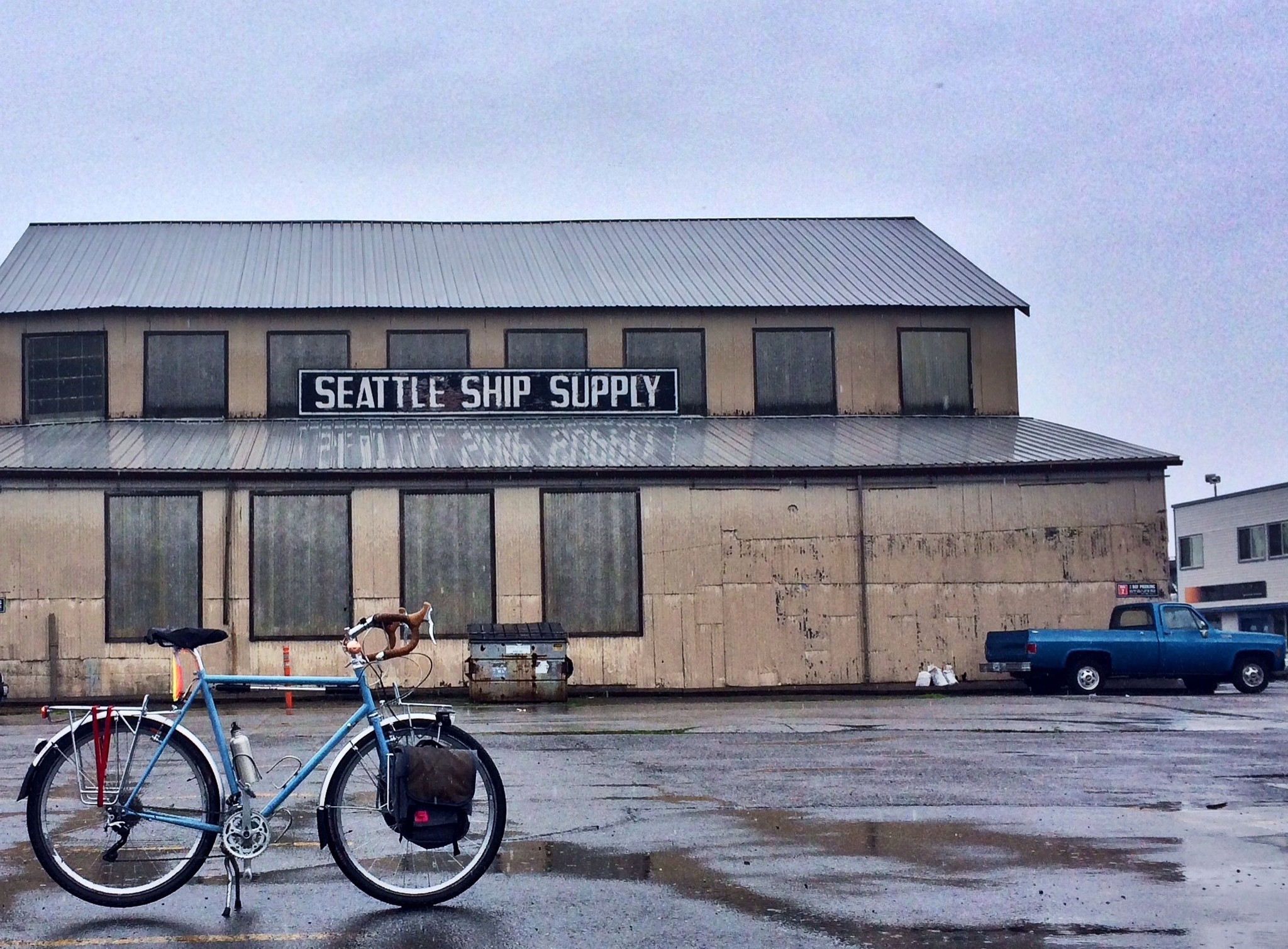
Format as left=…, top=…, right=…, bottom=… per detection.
left=377, top=741, right=478, bottom=849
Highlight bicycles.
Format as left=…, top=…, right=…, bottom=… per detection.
left=16, top=600, right=510, bottom=919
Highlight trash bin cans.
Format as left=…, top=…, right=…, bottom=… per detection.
left=467, top=622, right=569, bottom=703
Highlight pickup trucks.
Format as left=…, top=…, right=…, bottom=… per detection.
left=976, top=601, right=1288, bottom=697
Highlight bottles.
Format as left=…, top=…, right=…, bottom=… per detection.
left=229, top=721, right=259, bottom=784
